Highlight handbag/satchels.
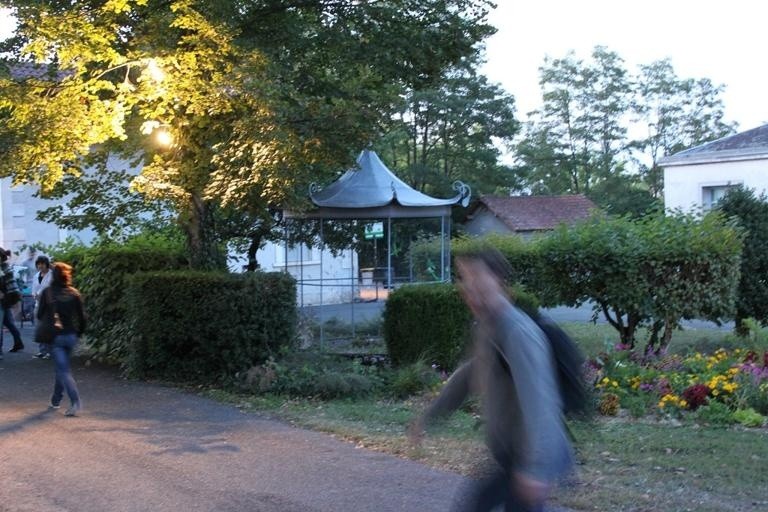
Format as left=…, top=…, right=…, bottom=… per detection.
left=33, top=313, right=63, bottom=343
left=528, top=304, right=587, bottom=414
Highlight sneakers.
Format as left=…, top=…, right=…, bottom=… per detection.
left=32, top=352, right=50, bottom=360
left=48, top=400, right=60, bottom=408
left=10, top=343, right=24, bottom=352
left=65, top=402, right=82, bottom=416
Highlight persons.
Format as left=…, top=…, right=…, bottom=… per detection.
left=30, top=255, right=56, bottom=360
left=36, top=261, right=87, bottom=417
left=0, top=248, right=26, bottom=359
left=405, top=241, right=597, bottom=511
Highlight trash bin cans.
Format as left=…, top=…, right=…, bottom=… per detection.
left=360, top=267, right=395, bottom=289
